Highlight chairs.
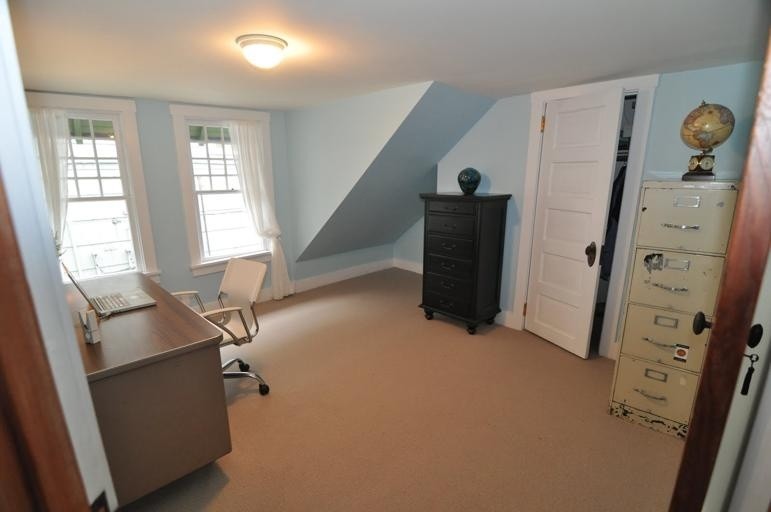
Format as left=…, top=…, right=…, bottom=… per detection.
left=169, top=255, right=270, bottom=401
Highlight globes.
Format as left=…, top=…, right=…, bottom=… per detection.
left=680, top=100, right=735, bottom=180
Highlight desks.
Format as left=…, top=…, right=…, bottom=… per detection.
left=62, top=270, right=234, bottom=510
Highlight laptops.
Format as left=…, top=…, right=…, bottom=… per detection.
left=62, top=260, right=157, bottom=320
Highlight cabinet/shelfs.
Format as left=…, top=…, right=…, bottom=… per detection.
left=604, top=180, right=743, bottom=442
left=418, top=191, right=514, bottom=335
